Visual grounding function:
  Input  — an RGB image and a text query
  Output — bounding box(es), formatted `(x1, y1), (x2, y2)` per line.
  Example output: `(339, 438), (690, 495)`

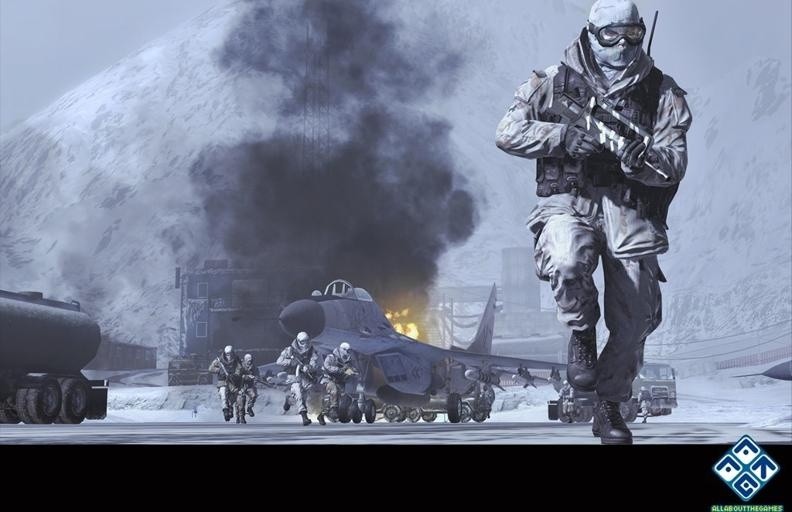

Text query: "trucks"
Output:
(552, 362), (677, 428)
(0, 288), (101, 425)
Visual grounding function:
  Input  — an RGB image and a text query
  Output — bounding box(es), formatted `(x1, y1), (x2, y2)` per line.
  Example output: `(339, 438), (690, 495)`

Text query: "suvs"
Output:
(168, 359), (212, 387)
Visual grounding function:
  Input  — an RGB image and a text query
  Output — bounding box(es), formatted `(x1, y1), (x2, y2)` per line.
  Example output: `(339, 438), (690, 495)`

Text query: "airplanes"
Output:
(254, 279), (571, 424)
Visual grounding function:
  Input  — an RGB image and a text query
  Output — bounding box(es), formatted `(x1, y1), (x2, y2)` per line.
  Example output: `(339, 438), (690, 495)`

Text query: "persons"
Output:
(318, 343), (360, 425)
(238, 354), (260, 423)
(558, 379), (574, 423)
(275, 332), (319, 425)
(492, 0), (692, 444)
(520, 366), (537, 388)
(210, 345), (244, 424)
(638, 386), (652, 424)
